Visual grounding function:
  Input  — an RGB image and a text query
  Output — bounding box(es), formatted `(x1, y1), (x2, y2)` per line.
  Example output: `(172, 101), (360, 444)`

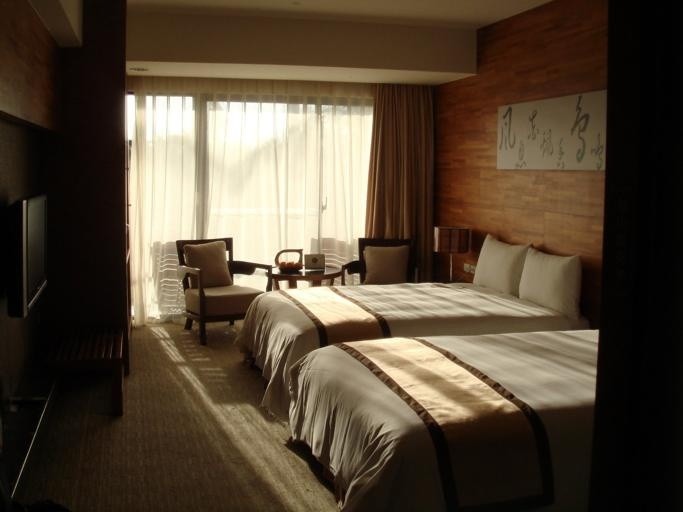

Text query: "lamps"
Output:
(433, 227), (470, 282)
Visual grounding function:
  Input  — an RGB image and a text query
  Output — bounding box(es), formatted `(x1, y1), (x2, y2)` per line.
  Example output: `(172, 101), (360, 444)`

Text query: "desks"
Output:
(24, 330), (124, 418)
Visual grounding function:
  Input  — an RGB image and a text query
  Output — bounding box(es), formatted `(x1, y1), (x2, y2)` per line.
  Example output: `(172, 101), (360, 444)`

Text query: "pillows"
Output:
(363, 245), (410, 284)
(182, 240), (233, 288)
(473, 234), (583, 323)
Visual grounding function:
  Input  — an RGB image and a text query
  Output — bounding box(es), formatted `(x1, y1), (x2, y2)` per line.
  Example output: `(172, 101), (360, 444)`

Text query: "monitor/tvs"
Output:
(4, 192), (47, 317)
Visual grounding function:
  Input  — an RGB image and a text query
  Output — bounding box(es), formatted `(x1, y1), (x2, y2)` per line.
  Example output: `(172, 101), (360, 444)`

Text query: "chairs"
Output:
(342, 238), (418, 286)
(176, 238), (272, 345)
